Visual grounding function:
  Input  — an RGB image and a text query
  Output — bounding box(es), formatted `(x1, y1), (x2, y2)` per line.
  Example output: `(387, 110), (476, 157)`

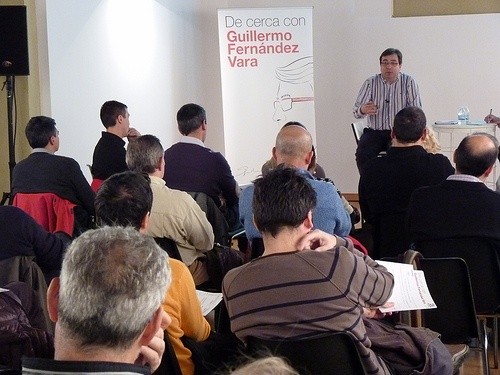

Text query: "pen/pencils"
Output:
(487, 108), (492, 123)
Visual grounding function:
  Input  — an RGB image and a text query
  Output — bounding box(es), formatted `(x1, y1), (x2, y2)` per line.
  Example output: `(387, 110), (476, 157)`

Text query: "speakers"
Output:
(0, 5), (29, 75)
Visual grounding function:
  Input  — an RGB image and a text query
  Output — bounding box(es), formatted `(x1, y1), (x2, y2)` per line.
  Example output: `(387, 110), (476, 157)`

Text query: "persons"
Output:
(227, 356), (300, 375)
(21, 226), (171, 374)
(407, 132), (500, 314)
(308, 146), (360, 225)
(94, 174), (212, 375)
(10, 116), (97, 237)
(221, 163), (469, 374)
(353, 48), (425, 174)
(0, 205), (74, 336)
(239, 125), (351, 259)
(127, 134), (248, 289)
(261, 121), (326, 179)
(485, 115), (500, 127)
(91, 100), (141, 183)
(358, 105), (456, 259)
(163, 104), (249, 253)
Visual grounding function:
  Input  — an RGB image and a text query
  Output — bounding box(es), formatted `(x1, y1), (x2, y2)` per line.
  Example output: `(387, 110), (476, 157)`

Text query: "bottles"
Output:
(458, 106), (466, 126)
(464, 106), (470, 125)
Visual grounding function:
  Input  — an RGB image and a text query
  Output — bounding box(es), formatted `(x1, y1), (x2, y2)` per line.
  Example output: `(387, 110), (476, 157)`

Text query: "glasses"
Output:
(380, 61), (399, 67)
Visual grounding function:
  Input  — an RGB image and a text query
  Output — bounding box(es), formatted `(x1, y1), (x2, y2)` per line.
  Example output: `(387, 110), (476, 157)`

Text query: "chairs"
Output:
(73, 183), (500, 375)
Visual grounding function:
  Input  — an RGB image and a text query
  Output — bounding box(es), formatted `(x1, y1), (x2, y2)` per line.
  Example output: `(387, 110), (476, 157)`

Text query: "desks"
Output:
(433, 121), (497, 191)
